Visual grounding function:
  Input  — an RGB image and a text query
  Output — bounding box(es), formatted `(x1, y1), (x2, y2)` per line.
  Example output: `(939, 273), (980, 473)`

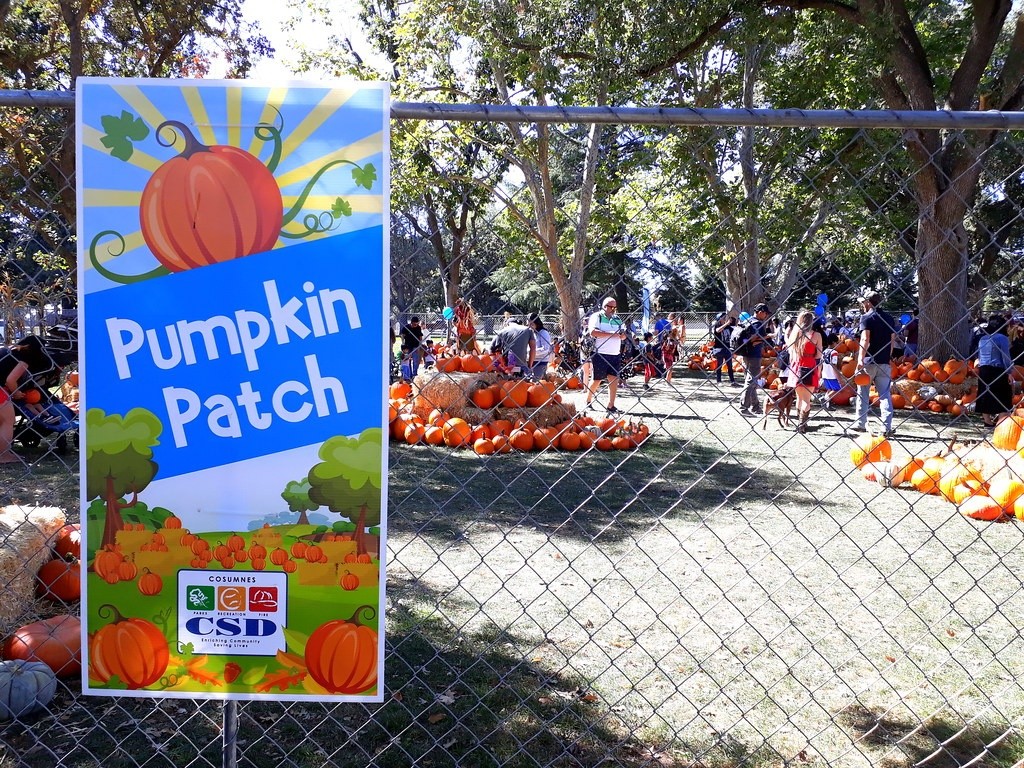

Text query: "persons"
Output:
(787, 312), (822, 433)
(849, 291), (896, 437)
(971, 315), (1024, 367)
(713, 311), (740, 386)
(820, 333), (841, 411)
(581, 297), (685, 414)
(978, 315), (1014, 428)
(525, 313), (551, 382)
(402, 317), (435, 376)
(893, 310), (918, 359)
(740, 303), (776, 416)
(491, 318), (536, 378)
(768, 317), (797, 345)
(0, 335), (61, 463)
(446, 310), (481, 358)
(812, 315), (854, 337)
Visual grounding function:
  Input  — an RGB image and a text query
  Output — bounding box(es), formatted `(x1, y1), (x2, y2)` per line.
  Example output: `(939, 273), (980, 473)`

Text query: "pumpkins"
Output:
(65, 369), (79, 387)
(23, 389), (41, 404)
(687, 340), (1024, 522)
(0, 523), (82, 722)
(389, 341), (649, 451)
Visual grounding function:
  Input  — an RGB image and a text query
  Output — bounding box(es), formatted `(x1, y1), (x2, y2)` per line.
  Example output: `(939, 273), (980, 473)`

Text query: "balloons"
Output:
(740, 312), (750, 321)
(443, 308), (452, 320)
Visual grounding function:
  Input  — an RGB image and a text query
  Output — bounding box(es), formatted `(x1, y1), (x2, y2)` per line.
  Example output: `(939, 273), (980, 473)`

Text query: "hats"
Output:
(754, 303), (772, 314)
(739, 312), (750, 322)
(716, 313), (726, 321)
(857, 291), (879, 303)
(653, 312), (660, 319)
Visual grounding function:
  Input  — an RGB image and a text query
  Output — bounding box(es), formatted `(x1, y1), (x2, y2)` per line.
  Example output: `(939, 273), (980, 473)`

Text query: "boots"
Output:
(796, 407), (811, 432)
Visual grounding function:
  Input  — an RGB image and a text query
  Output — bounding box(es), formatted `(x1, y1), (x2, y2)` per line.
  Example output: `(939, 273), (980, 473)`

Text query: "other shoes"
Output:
(642, 384), (653, 391)
(850, 426), (866, 432)
(882, 428), (895, 438)
(731, 382), (741, 388)
(587, 402), (593, 410)
(741, 409), (756, 417)
(607, 406), (624, 414)
(817, 392), (826, 407)
(39, 413), (60, 425)
(717, 381), (724, 386)
(0, 453), (24, 463)
(752, 407), (763, 414)
(984, 422), (995, 427)
(824, 406), (836, 411)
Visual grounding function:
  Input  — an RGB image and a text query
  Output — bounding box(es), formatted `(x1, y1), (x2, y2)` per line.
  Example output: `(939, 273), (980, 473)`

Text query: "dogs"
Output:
(763, 386), (796, 430)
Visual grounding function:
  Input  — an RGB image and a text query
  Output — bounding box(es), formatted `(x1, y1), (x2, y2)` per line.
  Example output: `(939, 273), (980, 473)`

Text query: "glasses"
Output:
(606, 305), (617, 310)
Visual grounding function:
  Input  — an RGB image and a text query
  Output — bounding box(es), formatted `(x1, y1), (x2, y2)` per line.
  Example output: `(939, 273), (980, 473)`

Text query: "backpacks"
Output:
(777, 350), (790, 371)
(729, 317), (761, 355)
